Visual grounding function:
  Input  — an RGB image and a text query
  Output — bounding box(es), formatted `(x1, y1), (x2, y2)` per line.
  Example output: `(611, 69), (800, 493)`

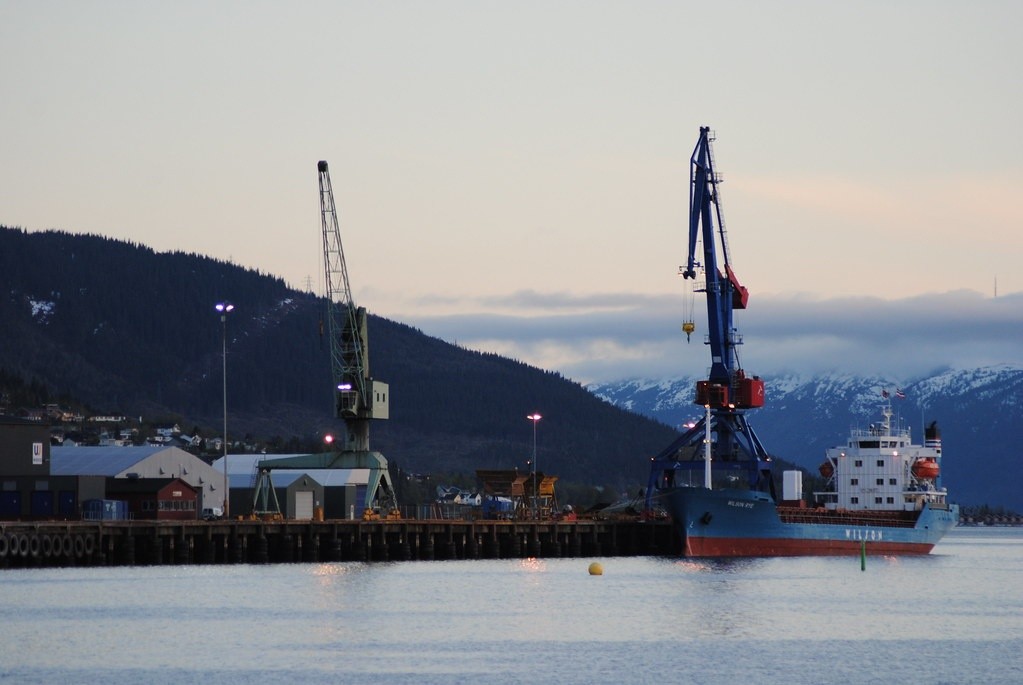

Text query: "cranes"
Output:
(245, 159), (403, 522)
(645, 124), (777, 518)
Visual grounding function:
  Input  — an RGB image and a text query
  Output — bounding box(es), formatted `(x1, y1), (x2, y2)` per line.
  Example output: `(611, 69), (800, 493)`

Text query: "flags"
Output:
(896, 388), (906, 400)
(881, 390), (890, 399)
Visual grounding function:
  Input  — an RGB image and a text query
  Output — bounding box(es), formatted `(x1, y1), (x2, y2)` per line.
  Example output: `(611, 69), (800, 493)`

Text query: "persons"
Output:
(562, 502), (574, 515)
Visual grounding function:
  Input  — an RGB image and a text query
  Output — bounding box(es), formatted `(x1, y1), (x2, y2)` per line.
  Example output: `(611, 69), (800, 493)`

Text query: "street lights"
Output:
(216, 303), (235, 521)
(526, 415), (541, 508)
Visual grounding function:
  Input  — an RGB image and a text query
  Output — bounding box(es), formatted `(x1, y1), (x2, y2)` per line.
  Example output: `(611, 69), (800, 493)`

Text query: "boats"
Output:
(913, 458), (939, 479)
(677, 386), (961, 560)
(819, 461), (838, 479)
